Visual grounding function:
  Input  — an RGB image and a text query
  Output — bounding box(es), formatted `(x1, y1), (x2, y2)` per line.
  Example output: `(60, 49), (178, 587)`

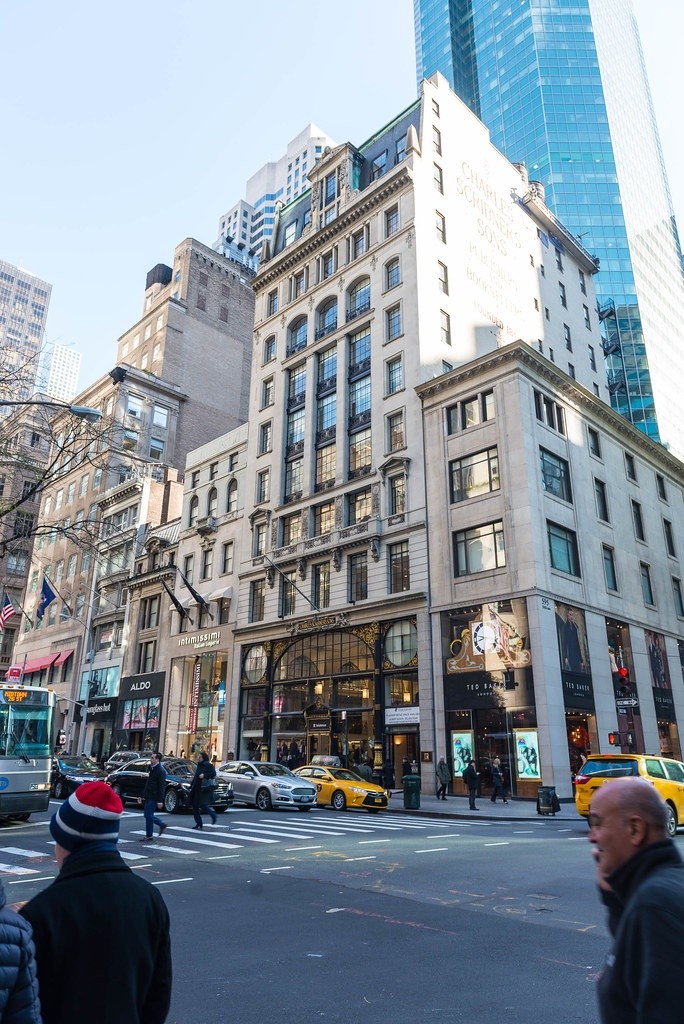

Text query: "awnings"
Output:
(169, 587), (233, 611)
(4, 649), (74, 677)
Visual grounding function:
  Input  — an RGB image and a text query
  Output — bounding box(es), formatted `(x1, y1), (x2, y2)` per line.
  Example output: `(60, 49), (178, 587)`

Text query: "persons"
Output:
(180, 745), (183, 757)
(132, 698), (160, 723)
(60, 748), (109, 769)
(16, 780), (172, 1024)
(137, 752), (168, 842)
(649, 631), (669, 689)
(0, 868), (42, 1024)
(246, 737), (258, 756)
(189, 751), (218, 829)
(276, 752), (294, 771)
(281, 737), (307, 759)
(588, 776), (684, 1024)
(522, 741), (538, 775)
(562, 607), (585, 673)
(382, 756), (509, 811)
(457, 743), (471, 772)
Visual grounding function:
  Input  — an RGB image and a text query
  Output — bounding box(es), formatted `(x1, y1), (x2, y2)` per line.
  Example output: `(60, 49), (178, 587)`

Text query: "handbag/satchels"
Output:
(502, 778), (510, 794)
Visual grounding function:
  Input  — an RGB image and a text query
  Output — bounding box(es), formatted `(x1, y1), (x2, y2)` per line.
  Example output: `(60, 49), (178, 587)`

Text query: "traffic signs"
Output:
(616, 698), (638, 716)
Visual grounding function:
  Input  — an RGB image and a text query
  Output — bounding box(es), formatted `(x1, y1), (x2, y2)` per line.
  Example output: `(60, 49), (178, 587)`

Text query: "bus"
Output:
(0, 682), (56, 823)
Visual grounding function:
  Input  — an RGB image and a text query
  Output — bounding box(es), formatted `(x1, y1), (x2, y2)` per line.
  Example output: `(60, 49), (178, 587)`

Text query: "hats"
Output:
(49, 783), (124, 852)
(440, 756), (445, 761)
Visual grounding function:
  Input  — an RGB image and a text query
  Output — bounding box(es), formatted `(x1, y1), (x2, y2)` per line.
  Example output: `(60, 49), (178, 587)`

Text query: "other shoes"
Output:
(442, 797), (448, 801)
(491, 798), (496, 803)
(139, 836), (153, 842)
(503, 799), (508, 804)
(470, 807), (480, 811)
(436, 793), (440, 800)
(158, 824), (168, 836)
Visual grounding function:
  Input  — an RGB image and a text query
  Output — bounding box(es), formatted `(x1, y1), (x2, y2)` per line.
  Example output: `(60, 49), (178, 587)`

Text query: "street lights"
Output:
(60, 614), (93, 756)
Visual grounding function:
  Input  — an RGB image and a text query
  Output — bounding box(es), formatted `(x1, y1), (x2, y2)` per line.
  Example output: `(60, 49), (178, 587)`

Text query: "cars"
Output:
(51, 757), (108, 795)
(214, 761), (318, 812)
(104, 755), (234, 814)
(289, 755), (389, 814)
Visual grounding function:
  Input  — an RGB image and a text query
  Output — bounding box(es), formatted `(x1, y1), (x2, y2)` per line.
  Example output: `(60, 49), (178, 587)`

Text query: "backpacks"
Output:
(463, 769), (470, 785)
(201, 766), (219, 791)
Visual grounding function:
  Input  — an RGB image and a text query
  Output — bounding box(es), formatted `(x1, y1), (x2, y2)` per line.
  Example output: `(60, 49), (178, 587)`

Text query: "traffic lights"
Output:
(609, 733), (620, 745)
(625, 734), (634, 744)
(619, 666), (631, 695)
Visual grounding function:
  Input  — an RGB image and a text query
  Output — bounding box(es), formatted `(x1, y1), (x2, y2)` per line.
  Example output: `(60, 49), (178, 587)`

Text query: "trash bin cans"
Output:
(401, 775), (421, 809)
(537, 786), (555, 816)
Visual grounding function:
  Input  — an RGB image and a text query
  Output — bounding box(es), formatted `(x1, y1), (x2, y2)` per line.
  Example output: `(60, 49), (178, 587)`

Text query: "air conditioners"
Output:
(195, 517), (215, 535)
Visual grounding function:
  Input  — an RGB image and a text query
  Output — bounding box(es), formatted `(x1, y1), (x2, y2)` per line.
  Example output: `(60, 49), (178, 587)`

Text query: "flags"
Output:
(0, 589), (17, 632)
(34, 575), (56, 631)
(158, 572), (205, 618)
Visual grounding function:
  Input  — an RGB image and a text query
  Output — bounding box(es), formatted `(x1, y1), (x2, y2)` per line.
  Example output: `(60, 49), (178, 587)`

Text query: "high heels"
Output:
(192, 823), (202, 829)
(211, 813), (218, 825)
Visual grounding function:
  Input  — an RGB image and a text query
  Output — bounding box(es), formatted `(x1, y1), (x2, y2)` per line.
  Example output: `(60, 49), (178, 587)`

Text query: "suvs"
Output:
(575, 753), (684, 836)
(104, 750), (154, 773)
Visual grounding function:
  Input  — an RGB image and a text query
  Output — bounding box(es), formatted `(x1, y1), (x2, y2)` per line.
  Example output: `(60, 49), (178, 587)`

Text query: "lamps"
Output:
(349, 600), (356, 606)
(278, 615), (284, 620)
